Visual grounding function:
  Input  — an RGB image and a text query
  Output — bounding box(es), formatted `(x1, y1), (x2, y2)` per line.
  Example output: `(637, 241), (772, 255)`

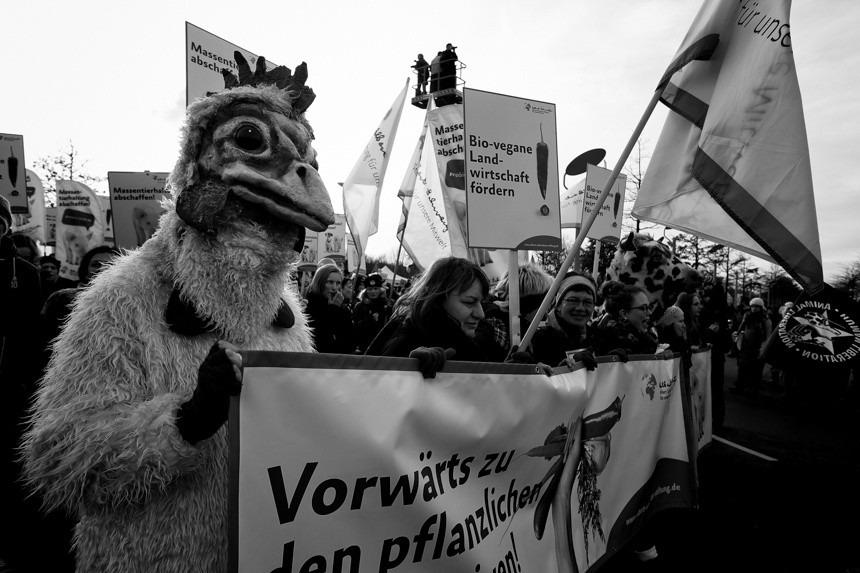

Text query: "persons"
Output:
(365, 256), (534, 379)
(18, 51), (336, 573)
(531, 271), (598, 371)
(0, 196), (124, 383)
(307, 257), (411, 356)
(483, 262), (554, 362)
(594, 273), (794, 428)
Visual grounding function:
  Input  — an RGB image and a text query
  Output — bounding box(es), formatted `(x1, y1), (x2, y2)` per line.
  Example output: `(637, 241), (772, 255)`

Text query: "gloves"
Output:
(609, 348), (631, 364)
(409, 346), (456, 377)
(574, 346), (598, 371)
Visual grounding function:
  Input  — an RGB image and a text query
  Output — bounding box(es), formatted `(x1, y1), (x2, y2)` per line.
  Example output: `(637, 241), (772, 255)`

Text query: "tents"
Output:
(369, 266), (408, 288)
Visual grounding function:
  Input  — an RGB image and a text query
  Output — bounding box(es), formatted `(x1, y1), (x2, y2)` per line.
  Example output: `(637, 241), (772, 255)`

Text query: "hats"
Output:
(0, 195), (12, 227)
(556, 275), (596, 307)
(750, 298), (764, 307)
(364, 274), (382, 288)
(653, 306), (684, 327)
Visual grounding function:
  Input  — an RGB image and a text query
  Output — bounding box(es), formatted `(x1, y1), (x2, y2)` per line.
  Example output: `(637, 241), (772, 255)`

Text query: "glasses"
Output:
(632, 304), (651, 315)
(562, 298), (593, 307)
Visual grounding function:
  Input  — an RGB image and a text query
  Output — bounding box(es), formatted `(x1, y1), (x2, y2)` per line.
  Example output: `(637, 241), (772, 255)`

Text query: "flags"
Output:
(630, 0), (825, 300)
(338, 77), (413, 267)
(397, 98), (494, 271)
